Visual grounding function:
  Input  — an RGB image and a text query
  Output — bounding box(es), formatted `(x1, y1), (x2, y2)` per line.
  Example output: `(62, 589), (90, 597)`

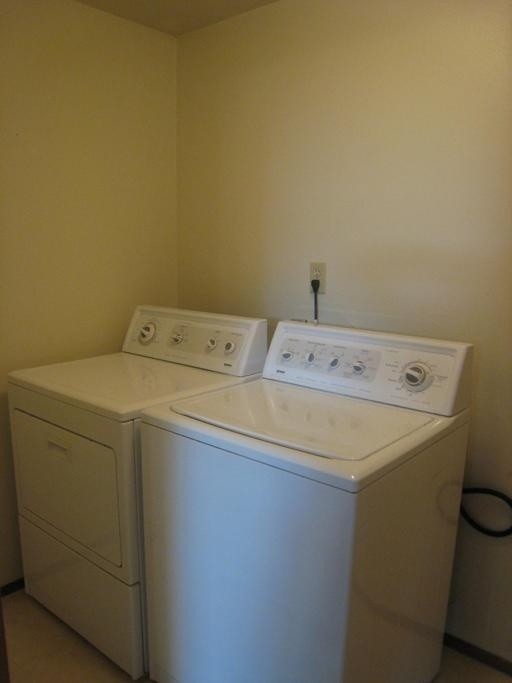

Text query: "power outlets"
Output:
(309, 263), (323, 290)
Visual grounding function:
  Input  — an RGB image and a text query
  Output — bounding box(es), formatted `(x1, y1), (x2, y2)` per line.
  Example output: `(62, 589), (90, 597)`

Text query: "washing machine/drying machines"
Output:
(140, 319), (476, 683)
(7, 306), (269, 683)
